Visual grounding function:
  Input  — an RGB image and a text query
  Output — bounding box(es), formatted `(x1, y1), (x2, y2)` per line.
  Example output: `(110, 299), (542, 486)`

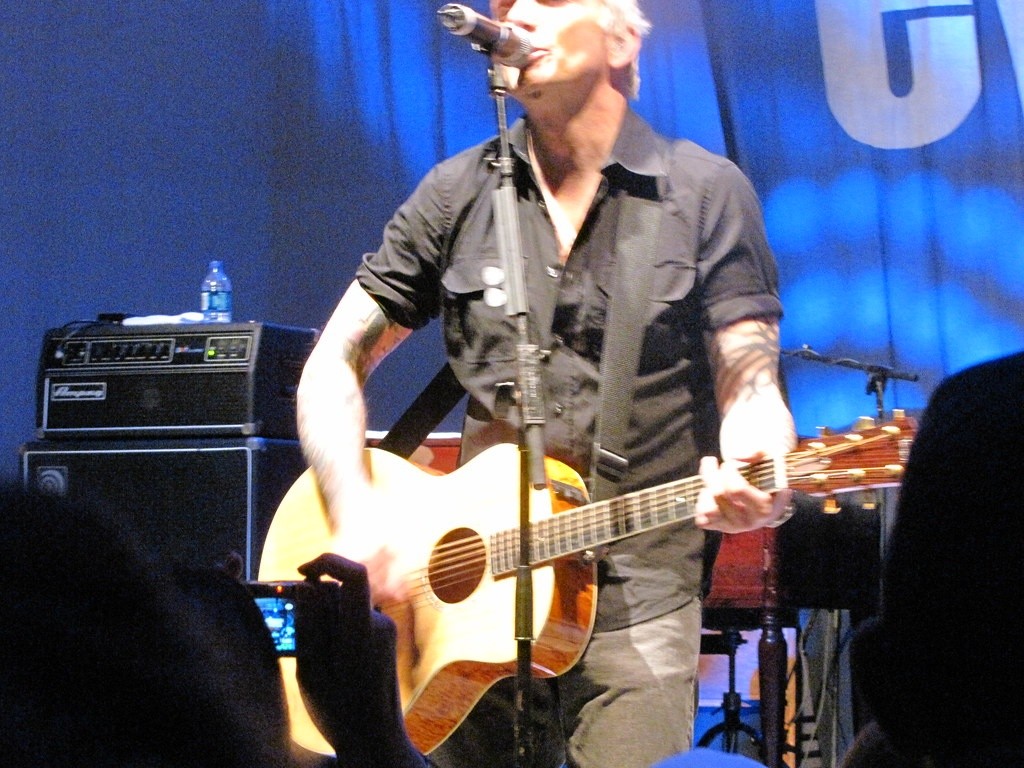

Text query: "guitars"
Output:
(251, 404), (916, 763)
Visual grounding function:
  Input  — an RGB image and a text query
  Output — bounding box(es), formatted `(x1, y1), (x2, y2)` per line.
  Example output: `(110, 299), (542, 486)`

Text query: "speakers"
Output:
(13, 437), (308, 768)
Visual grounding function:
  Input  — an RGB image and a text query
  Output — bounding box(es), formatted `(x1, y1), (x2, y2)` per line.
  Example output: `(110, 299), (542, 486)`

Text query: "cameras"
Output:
(231, 577), (341, 656)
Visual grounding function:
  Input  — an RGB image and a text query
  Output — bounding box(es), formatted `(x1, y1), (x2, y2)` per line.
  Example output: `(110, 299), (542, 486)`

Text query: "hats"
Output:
(0, 477), (289, 768)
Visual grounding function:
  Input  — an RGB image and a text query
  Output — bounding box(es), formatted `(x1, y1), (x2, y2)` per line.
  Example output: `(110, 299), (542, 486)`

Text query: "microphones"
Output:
(435, 3), (531, 68)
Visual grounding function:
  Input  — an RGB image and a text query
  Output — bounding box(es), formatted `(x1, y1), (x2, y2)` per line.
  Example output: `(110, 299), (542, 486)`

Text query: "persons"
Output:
(0, 476), (438, 768)
(294, 0), (800, 768)
(831, 350), (1024, 768)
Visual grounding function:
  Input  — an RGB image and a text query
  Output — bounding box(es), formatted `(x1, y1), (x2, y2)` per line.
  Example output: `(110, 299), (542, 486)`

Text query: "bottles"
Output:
(201, 261), (233, 323)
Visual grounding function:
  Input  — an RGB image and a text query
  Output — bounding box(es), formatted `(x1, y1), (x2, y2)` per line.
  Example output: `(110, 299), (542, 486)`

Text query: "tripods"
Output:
(696, 627), (768, 762)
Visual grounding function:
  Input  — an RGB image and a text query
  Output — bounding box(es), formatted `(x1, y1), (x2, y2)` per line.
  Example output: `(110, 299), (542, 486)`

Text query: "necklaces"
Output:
(526, 133), (572, 247)
